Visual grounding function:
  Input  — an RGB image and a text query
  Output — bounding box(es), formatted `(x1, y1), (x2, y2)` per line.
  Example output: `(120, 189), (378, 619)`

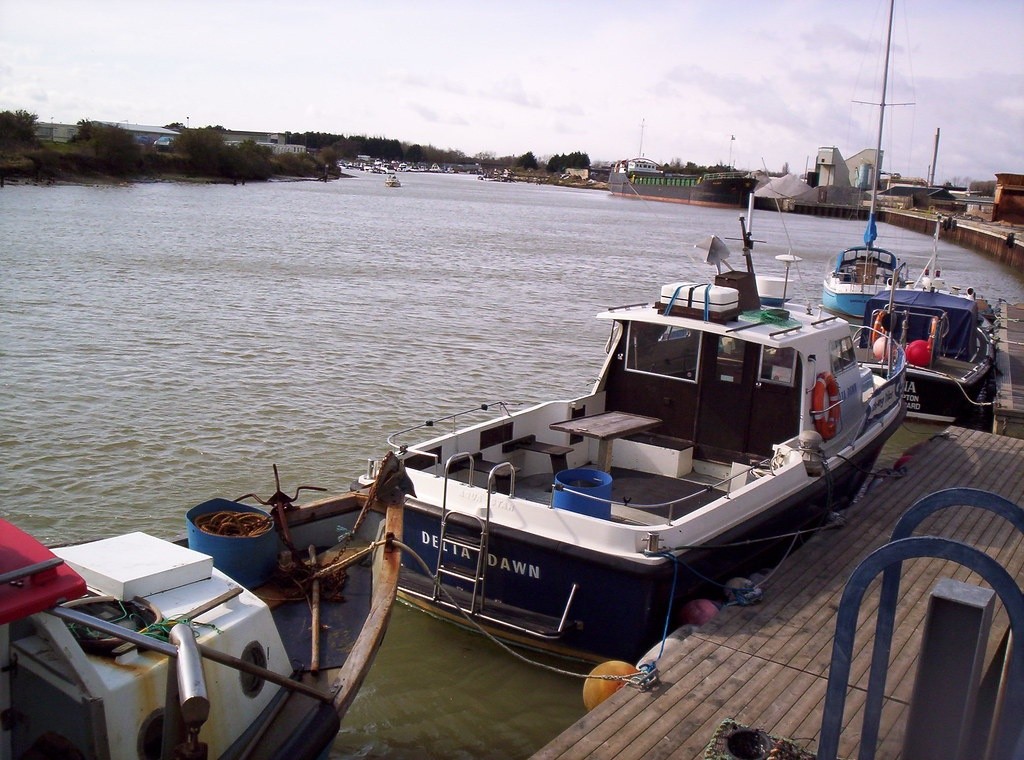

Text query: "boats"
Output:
(355, 190), (910, 668)
(0, 450), (417, 760)
(850, 213), (999, 429)
(385, 175), (402, 188)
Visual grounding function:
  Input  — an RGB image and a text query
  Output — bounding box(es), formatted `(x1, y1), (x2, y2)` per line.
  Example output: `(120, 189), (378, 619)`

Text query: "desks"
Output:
(549, 410), (662, 475)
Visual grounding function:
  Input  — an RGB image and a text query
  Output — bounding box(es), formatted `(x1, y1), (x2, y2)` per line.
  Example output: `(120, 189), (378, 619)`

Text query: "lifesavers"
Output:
(872, 311), (895, 345)
(929, 317), (938, 349)
(812, 372), (842, 440)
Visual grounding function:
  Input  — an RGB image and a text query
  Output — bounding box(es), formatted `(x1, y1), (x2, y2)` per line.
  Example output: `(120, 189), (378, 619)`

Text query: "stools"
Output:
(517, 440), (574, 480)
(463, 459), (521, 496)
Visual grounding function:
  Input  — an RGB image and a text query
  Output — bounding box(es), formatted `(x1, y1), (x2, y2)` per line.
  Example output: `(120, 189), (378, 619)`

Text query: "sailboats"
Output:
(821, 0), (913, 320)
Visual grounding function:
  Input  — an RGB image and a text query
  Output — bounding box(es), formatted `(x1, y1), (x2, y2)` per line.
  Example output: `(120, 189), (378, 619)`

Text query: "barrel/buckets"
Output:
(185, 498), (278, 591)
(552, 469), (613, 522)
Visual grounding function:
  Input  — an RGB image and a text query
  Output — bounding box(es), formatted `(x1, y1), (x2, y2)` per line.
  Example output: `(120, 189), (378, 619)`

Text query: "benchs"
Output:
(588, 432), (694, 479)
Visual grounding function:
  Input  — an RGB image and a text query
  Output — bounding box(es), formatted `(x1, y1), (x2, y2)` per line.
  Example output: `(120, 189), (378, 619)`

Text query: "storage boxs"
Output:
(661, 281), (740, 312)
